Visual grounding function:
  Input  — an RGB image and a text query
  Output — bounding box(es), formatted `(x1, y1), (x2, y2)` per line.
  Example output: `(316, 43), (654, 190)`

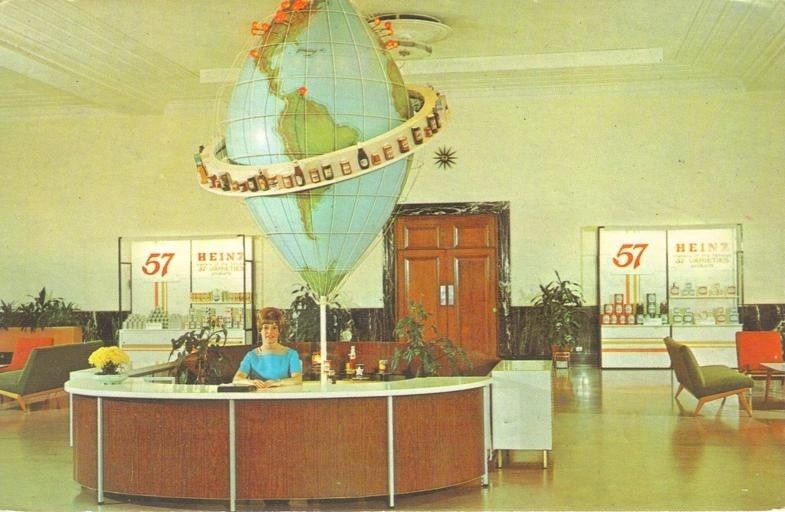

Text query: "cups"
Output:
(344, 362), (366, 379)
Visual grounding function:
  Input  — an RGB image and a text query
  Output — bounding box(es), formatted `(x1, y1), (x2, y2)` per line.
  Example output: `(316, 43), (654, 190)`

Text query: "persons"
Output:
(232, 306), (303, 388)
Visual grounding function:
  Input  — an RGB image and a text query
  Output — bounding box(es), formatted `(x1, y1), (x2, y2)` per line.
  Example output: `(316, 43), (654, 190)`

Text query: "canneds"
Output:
(207, 173), (294, 192)
(383, 145), (394, 160)
(397, 137), (410, 153)
(424, 114), (438, 137)
(309, 168), (320, 183)
(355, 363), (364, 379)
(601, 293), (667, 325)
(411, 126), (423, 144)
(339, 160), (352, 175)
(321, 164), (333, 180)
(378, 358), (389, 374)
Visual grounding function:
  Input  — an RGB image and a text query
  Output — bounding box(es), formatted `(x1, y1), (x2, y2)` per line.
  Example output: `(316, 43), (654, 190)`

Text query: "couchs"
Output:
(663, 336), (756, 417)
(0, 340), (104, 414)
(0, 337), (54, 372)
(735, 331), (785, 393)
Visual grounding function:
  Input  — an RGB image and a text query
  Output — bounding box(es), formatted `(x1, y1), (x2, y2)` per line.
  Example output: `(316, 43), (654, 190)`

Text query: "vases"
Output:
(94, 374), (128, 386)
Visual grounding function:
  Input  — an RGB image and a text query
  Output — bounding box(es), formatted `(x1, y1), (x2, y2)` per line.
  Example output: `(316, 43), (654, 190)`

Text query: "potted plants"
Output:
(530, 270), (587, 368)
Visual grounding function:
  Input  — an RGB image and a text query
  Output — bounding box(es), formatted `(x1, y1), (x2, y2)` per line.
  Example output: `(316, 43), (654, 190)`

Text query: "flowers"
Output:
(88, 346), (129, 373)
